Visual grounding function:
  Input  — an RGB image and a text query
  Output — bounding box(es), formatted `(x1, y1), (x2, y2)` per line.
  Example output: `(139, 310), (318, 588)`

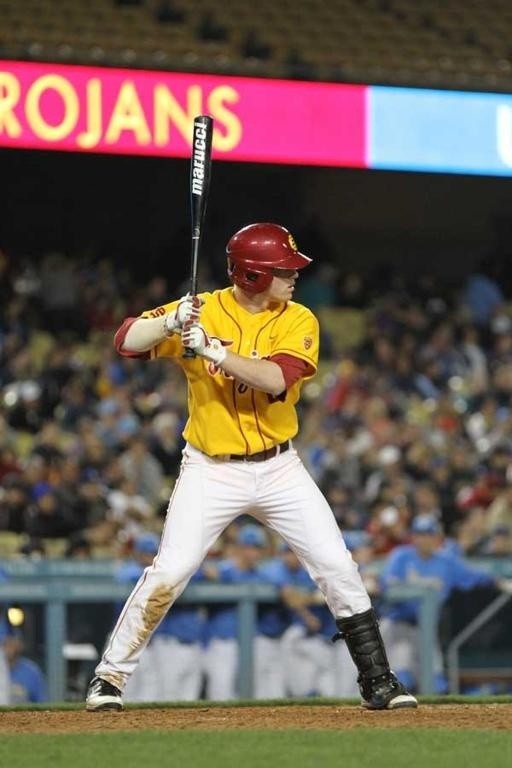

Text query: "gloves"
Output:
(182, 322), (227, 367)
(163, 294), (206, 339)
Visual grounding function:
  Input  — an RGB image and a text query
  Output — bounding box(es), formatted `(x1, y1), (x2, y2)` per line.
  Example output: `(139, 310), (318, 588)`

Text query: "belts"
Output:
(199, 438), (292, 463)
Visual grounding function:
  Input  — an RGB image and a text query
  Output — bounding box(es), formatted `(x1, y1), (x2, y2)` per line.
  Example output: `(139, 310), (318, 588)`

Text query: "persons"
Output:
(83, 223), (419, 711)
(3, 230), (509, 704)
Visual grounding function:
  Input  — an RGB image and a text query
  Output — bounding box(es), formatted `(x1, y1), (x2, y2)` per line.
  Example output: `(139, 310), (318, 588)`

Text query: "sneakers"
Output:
(361, 670), (420, 713)
(84, 676), (127, 711)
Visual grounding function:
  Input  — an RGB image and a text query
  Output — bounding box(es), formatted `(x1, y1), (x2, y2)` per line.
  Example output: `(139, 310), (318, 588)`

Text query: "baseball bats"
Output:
(183, 116), (213, 358)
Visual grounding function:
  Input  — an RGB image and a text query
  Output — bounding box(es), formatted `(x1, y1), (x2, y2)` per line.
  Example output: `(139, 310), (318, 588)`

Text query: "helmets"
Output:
(226, 222), (314, 294)
